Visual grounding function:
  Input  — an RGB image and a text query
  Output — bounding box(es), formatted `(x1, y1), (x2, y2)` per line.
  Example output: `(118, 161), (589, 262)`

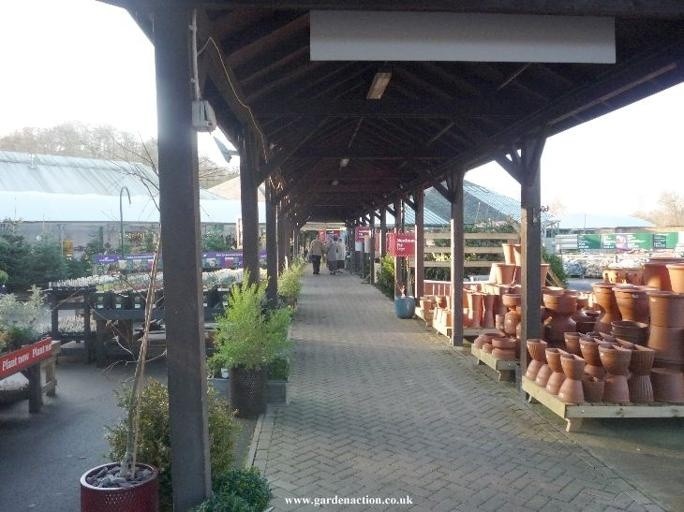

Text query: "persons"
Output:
(220, 234), (236, 250)
(289, 234), (349, 276)
(257, 231), (266, 249)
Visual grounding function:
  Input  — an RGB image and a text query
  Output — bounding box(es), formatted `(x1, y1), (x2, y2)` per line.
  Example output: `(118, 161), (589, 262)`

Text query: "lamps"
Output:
(366, 69), (391, 99)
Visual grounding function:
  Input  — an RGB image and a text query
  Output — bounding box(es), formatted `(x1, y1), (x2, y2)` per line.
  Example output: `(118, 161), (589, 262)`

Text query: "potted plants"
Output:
(277, 266), (302, 309)
(207, 265), (297, 418)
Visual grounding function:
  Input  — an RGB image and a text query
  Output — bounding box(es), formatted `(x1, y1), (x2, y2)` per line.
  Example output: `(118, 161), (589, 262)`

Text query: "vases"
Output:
(394, 297), (416, 318)
(81, 462), (160, 512)
